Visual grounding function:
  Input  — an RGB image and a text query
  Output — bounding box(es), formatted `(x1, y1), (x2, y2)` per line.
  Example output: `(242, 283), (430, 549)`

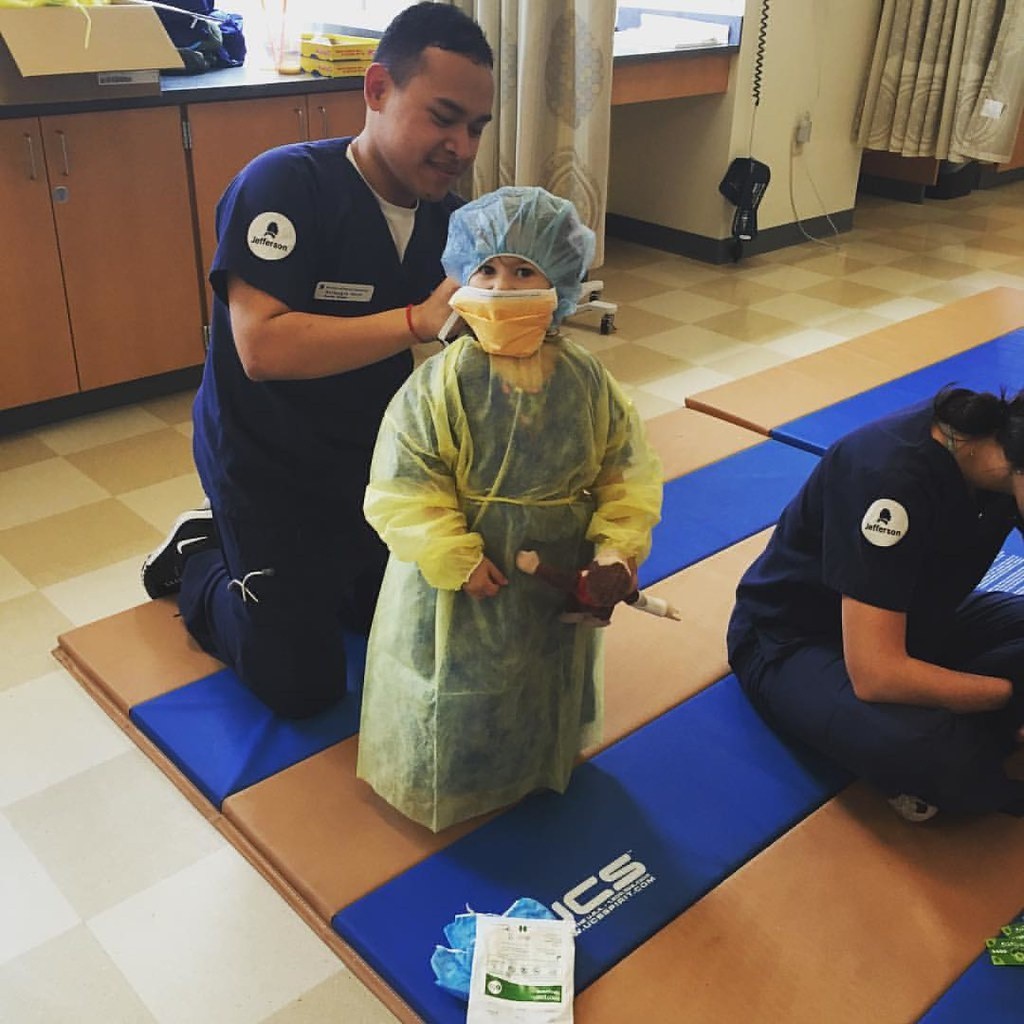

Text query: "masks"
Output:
(446, 284), (557, 358)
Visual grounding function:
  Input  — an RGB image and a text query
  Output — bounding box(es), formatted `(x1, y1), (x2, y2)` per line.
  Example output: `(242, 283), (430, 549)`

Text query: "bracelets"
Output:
(406, 303), (427, 345)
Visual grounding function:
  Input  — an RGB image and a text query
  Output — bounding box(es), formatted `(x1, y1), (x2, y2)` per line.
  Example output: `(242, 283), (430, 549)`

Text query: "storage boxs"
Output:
(0, 0), (185, 106)
(300, 33), (381, 78)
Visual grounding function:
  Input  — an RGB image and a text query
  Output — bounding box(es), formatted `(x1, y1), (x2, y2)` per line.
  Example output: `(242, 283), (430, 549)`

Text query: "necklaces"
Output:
(945, 425), (957, 451)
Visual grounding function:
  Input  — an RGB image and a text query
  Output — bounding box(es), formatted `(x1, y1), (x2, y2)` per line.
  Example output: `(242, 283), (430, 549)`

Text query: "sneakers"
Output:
(141, 508), (219, 600)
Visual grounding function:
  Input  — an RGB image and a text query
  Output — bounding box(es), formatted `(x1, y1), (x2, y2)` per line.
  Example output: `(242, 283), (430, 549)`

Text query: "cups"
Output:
(278, 37), (302, 76)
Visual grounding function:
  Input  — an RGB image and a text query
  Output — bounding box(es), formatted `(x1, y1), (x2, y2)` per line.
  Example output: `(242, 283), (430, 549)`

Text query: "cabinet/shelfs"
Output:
(0, 106), (207, 436)
(184, 89), (367, 339)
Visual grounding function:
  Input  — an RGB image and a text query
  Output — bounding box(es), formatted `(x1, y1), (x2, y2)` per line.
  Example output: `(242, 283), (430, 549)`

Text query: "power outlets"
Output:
(791, 124), (804, 156)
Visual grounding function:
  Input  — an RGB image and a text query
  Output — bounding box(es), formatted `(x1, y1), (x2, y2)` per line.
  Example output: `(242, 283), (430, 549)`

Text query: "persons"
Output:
(727, 382), (1024, 822)
(143, 1), (493, 714)
(362, 184), (663, 828)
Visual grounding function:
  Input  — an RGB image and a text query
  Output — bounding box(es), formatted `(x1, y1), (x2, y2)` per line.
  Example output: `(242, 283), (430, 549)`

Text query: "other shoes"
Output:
(888, 792), (938, 824)
